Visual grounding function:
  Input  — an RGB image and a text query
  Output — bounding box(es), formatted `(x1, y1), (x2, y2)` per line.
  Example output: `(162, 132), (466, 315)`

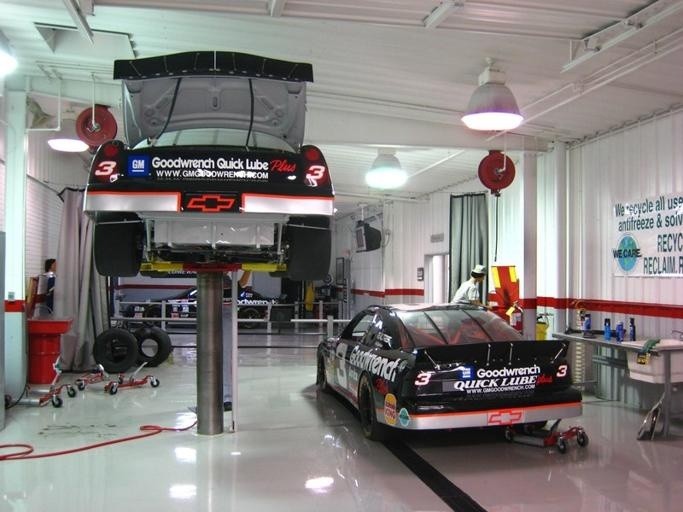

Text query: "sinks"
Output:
(622, 339), (683, 384)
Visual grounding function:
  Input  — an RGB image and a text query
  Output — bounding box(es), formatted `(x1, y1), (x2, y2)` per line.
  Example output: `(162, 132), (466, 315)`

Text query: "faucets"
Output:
(670, 329), (683, 341)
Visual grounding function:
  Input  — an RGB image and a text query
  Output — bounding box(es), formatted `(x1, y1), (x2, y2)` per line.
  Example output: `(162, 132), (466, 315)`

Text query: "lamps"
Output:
(461, 57), (524, 132)
(48, 109), (89, 152)
(366, 149), (407, 190)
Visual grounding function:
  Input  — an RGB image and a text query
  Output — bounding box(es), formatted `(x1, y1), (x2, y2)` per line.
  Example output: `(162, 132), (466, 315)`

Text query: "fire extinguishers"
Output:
(505, 301), (523, 335)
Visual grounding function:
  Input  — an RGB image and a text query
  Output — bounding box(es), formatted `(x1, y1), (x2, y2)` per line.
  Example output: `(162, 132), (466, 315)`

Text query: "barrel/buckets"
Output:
(28, 334), (60, 383)
(536, 315), (548, 340)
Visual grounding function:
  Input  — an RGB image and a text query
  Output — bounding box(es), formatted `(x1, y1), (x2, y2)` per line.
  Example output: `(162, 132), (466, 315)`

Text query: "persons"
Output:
(451, 264), (491, 310)
(44, 259), (56, 277)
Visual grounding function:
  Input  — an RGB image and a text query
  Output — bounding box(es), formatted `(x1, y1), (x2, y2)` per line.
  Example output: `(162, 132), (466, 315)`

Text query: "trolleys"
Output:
(0, 354), (76, 408)
(502, 415), (589, 455)
(74, 360), (160, 395)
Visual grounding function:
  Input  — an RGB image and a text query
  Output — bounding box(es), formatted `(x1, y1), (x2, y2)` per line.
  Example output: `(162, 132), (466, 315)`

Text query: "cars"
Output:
(315, 300), (581, 441)
(82, 49), (336, 282)
(123, 283), (277, 332)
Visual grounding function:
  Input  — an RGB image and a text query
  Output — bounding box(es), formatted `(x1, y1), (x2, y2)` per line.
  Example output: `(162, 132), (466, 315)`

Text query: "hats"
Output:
(472, 265), (488, 275)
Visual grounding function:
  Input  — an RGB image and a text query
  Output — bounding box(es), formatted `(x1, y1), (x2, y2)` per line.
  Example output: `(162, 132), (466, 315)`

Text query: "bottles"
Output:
(583, 313), (591, 333)
(629, 318), (636, 341)
(615, 321), (625, 343)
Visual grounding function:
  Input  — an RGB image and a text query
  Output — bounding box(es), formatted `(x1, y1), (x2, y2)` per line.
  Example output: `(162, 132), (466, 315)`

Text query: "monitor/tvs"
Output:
(355, 224), (380, 253)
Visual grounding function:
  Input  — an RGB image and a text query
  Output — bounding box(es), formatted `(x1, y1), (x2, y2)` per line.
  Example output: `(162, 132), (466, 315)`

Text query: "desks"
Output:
(551, 333), (683, 440)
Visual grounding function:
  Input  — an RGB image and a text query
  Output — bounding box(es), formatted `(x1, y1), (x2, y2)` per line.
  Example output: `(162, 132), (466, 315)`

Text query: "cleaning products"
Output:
(629, 318), (635, 341)
(616, 321), (623, 344)
